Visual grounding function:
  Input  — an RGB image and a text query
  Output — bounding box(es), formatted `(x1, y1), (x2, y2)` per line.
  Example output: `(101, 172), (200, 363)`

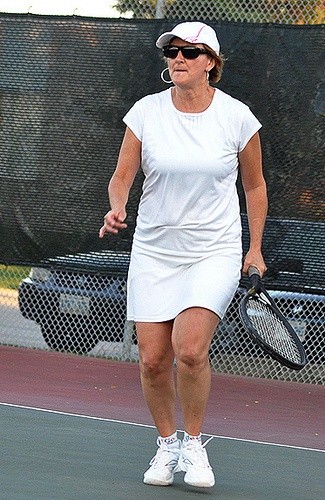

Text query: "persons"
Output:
(100, 20), (268, 488)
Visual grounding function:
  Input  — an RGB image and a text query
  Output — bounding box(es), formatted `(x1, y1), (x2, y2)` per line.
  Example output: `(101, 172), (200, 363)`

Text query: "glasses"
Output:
(161, 44), (213, 59)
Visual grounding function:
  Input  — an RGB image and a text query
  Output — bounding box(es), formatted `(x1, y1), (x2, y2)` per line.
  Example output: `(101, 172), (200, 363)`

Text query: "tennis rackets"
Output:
(239, 266), (307, 371)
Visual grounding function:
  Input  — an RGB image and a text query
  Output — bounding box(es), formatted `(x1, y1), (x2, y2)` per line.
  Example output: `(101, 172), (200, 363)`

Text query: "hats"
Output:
(156, 22), (220, 56)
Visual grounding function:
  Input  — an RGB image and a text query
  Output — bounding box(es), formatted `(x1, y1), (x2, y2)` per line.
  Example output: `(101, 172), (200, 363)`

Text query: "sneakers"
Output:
(179, 440), (215, 487)
(143, 440), (179, 486)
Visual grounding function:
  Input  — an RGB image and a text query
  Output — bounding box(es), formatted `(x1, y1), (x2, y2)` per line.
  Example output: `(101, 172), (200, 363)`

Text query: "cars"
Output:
(18, 214), (325, 364)
